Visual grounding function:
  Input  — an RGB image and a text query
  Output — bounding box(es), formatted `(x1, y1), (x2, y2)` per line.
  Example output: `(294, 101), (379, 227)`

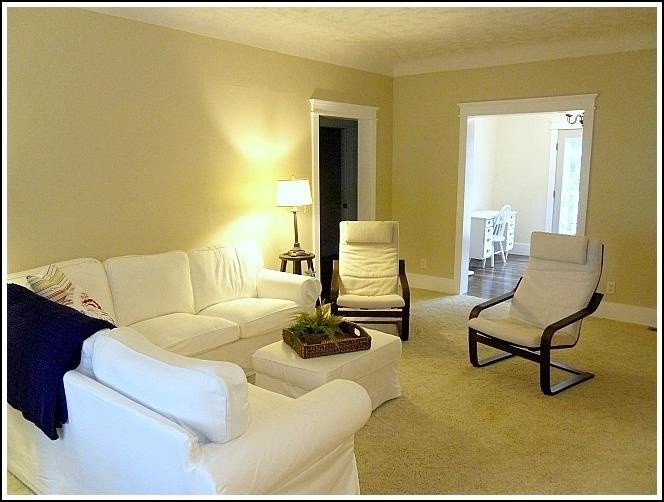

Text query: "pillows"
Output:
(26, 264), (116, 326)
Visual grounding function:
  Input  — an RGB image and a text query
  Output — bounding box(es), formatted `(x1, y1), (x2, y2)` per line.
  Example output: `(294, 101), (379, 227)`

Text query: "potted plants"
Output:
(283, 300), (346, 354)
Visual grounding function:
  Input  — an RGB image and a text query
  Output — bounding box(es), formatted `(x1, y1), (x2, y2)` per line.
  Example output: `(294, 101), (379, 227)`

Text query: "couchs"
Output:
(251, 326), (403, 414)
(7, 242), (322, 378)
(7, 283), (372, 493)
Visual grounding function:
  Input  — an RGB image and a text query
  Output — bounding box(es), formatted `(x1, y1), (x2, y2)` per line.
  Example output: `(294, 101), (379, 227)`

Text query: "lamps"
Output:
(274, 175), (314, 257)
(565, 111), (585, 127)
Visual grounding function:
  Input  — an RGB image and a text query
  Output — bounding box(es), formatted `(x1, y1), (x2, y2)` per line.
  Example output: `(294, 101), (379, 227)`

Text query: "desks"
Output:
(470, 210), (517, 268)
(279, 252), (321, 308)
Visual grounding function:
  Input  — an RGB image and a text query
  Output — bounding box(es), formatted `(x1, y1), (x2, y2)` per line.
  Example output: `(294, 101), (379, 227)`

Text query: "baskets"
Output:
(282, 321), (372, 358)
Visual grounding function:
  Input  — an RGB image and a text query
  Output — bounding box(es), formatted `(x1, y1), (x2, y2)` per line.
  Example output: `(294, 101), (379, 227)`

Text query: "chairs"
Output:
(466, 231), (605, 395)
(330, 220), (409, 340)
(491, 204), (511, 265)
